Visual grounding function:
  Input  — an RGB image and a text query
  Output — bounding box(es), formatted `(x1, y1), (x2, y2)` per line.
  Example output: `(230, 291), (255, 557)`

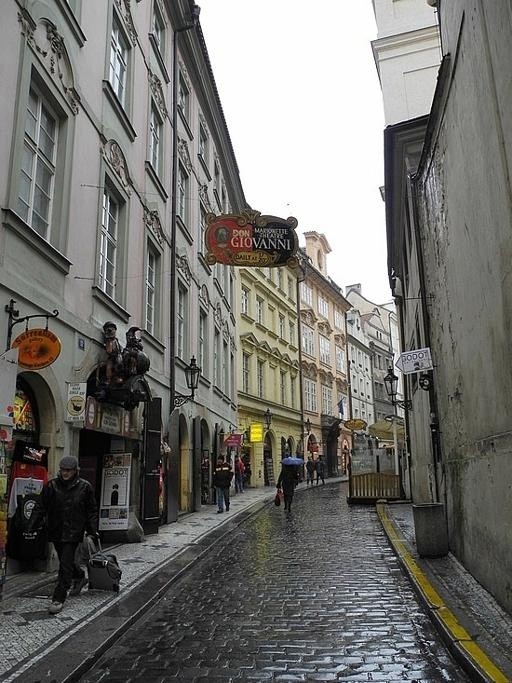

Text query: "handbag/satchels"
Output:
(274, 487), (282, 508)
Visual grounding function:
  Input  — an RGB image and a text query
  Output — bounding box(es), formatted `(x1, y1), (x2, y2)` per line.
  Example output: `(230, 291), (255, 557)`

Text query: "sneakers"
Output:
(48, 598), (64, 615)
(70, 577), (89, 597)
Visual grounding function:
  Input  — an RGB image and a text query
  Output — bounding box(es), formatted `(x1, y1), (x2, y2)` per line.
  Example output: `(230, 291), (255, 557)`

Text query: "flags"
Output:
(337, 400), (344, 416)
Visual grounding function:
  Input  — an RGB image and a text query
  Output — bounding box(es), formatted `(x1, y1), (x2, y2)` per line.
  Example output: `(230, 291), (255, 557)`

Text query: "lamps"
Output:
(383, 365), (413, 413)
(263, 407), (273, 430)
(303, 417), (313, 436)
(170, 355), (202, 407)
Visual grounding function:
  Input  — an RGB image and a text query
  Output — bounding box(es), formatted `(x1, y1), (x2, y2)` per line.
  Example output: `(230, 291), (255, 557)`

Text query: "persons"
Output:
(111, 484), (118, 506)
(28, 456), (99, 614)
(213, 455), (234, 513)
(235, 455), (245, 496)
(314, 457), (325, 485)
(276, 463), (298, 513)
(306, 456), (315, 484)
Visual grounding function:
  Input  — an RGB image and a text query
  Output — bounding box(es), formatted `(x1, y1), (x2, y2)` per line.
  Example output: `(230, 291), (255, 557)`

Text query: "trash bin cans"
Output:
(413, 503), (448, 559)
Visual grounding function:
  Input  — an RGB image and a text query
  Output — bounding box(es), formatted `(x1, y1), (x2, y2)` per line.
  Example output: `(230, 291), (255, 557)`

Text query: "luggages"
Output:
(85, 532), (121, 594)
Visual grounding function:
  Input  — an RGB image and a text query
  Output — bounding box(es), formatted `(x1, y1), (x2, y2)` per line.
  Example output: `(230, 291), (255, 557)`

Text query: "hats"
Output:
(59, 457), (78, 471)
